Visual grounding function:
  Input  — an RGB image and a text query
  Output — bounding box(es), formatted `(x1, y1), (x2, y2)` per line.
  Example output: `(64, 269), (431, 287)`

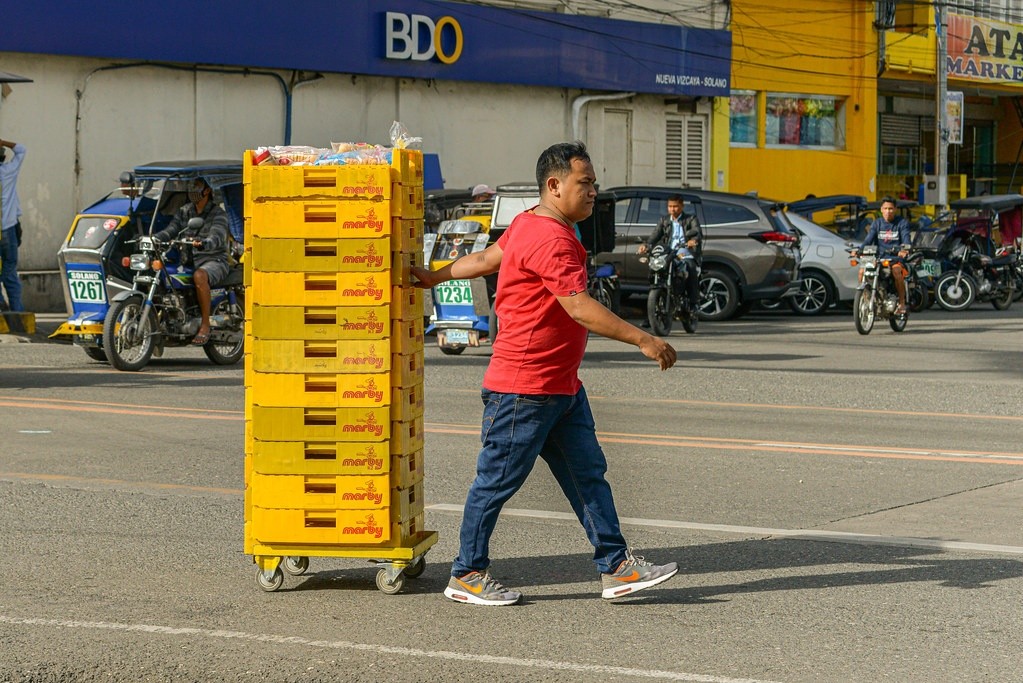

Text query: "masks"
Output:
(188, 190), (206, 205)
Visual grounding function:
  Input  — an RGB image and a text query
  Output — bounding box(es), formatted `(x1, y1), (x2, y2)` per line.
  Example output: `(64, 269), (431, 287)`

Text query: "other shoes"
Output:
(640, 319), (651, 329)
(690, 303), (700, 315)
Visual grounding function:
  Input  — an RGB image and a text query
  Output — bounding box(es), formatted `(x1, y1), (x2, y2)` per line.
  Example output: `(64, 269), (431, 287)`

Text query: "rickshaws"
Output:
(424, 182), (619, 355)
(48, 158), (244, 372)
(787, 193), (931, 253)
(908, 193), (1023, 312)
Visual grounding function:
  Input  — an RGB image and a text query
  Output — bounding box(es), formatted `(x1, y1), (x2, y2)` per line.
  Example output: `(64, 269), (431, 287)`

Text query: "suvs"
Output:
(596, 186), (802, 322)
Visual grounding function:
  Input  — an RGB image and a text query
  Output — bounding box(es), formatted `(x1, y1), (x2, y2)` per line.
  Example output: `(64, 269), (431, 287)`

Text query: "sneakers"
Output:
(601, 547), (680, 600)
(444, 564), (523, 606)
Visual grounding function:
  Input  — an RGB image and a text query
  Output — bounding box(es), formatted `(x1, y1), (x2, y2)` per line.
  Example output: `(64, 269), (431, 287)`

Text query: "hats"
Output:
(472, 184), (496, 196)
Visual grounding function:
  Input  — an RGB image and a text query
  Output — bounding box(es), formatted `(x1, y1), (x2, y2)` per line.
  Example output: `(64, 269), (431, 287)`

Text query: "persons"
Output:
(850, 198), (912, 314)
(464, 184), (497, 216)
(152, 177), (230, 345)
(0, 139), (26, 312)
(800, 193), (817, 221)
(573, 222), (581, 241)
(638, 194), (704, 327)
(410, 143), (679, 607)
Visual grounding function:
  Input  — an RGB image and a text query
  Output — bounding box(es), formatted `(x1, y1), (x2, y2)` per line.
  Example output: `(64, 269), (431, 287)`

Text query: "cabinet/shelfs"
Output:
(243, 149), (423, 547)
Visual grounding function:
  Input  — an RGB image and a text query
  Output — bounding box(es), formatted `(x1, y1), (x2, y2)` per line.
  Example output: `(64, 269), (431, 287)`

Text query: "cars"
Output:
(783, 211), (866, 316)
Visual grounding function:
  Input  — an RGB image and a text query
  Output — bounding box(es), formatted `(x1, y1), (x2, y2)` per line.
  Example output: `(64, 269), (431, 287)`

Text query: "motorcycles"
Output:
(634, 230), (698, 336)
(844, 237), (922, 334)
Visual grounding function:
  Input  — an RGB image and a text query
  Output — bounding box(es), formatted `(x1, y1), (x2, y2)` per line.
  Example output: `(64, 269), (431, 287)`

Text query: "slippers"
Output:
(894, 304), (911, 315)
(191, 327), (214, 346)
